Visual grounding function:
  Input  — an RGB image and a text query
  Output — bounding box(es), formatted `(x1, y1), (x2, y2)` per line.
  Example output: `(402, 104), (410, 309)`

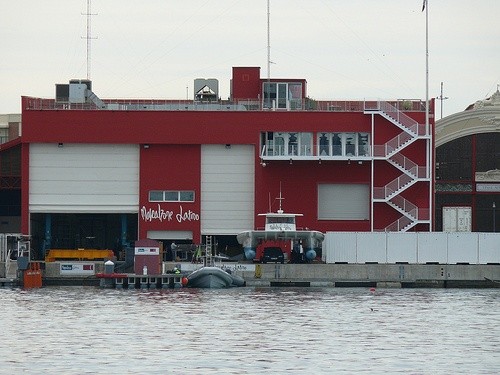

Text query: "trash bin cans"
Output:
(23, 261), (46, 290)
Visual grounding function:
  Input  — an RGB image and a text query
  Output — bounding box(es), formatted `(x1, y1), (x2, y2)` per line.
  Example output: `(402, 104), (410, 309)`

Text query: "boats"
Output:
(182, 234), (245, 290)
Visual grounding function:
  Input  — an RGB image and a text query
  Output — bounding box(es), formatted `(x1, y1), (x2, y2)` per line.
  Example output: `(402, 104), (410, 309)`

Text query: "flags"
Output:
(422, 0), (426, 12)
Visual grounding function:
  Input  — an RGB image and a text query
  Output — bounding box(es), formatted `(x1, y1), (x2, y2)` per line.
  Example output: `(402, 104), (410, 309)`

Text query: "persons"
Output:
(170, 240), (179, 263)
(190, 245), (202, 264)
